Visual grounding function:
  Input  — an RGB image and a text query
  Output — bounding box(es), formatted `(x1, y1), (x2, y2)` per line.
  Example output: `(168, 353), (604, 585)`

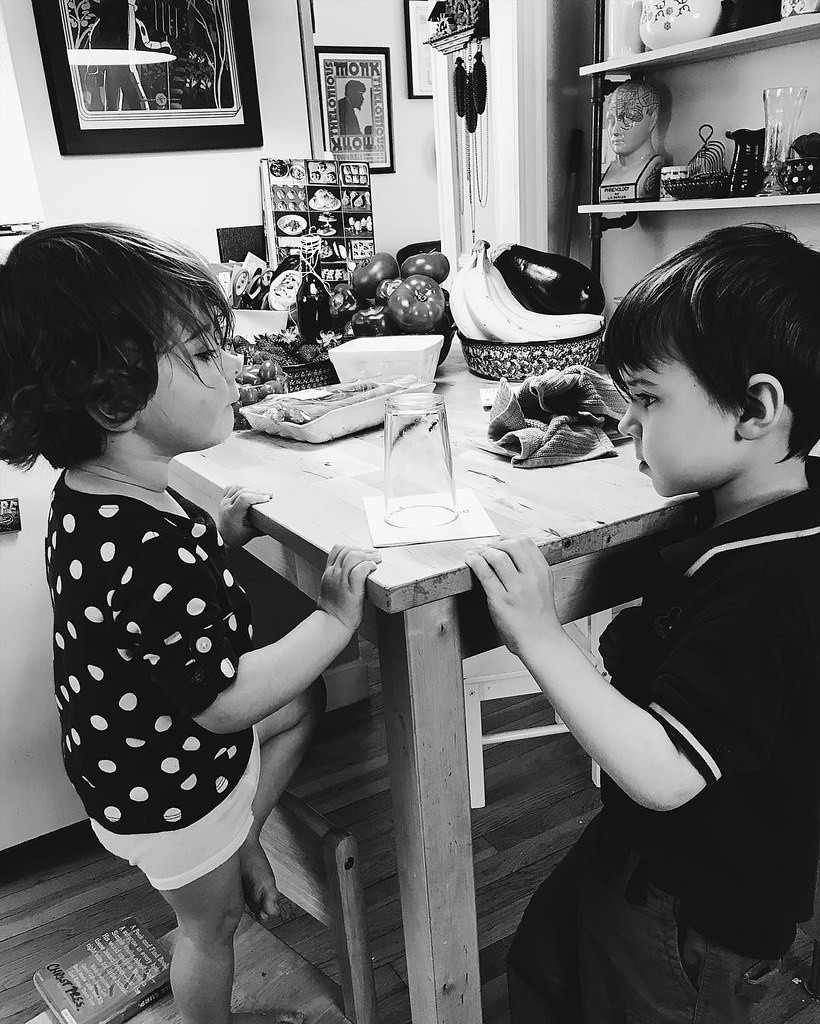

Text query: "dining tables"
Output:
(166, 336), (702, 1024)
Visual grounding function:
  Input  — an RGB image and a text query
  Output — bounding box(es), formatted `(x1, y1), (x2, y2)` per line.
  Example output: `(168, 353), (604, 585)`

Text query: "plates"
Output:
(309, 197), (342, 210)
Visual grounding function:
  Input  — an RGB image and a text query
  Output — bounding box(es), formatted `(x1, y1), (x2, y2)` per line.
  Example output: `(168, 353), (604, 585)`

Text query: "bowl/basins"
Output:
(328, 335), (444, 383)
(282, 359), (341, 393)
(277, 214), (307, 235)
(457, 321), (608, 383)
(421, 326), (456, 367)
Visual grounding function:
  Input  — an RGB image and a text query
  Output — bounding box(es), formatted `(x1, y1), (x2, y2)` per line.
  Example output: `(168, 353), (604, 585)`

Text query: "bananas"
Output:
(450, 240), (606, 339)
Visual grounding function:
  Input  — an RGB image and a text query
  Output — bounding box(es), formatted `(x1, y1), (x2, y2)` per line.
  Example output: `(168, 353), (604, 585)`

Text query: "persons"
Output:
(465, 222), (820, 1024)
(0, 223), (381, 1024)
(599, 81), (663, 202)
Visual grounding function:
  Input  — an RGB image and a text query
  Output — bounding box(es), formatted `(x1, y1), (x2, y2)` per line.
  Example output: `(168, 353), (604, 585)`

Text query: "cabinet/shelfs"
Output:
(578, 13), (820, 213)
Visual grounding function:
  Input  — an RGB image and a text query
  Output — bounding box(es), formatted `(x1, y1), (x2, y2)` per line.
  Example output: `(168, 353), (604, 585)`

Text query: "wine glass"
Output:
(755, 86), (808, 197)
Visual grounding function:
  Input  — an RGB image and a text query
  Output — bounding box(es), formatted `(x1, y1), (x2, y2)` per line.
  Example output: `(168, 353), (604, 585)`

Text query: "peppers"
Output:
(330, 278), (405, 335)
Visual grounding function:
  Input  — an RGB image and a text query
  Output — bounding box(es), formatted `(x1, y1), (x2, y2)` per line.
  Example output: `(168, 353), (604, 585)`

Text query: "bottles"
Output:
(296, 234), (331, 345)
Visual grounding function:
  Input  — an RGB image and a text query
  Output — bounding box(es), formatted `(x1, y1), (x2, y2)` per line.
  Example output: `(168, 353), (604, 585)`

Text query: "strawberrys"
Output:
(220, 330), (341, 371)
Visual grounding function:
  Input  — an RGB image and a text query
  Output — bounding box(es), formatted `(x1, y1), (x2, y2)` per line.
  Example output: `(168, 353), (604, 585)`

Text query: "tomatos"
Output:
(227, 361), (287, 402)
(388, 275), (445, 333)
(352, 252), (399, 299)
(401, 251), (449, 285)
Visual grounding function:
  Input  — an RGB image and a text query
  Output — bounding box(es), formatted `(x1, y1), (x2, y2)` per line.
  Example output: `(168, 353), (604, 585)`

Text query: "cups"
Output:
(776, 157), (820, 194)
(384, 393), (459, 529)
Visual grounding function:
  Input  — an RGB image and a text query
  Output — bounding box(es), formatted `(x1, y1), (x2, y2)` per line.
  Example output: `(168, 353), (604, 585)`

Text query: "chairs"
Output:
(24, 790), (380, 1024)
(462, 608), (612, 810)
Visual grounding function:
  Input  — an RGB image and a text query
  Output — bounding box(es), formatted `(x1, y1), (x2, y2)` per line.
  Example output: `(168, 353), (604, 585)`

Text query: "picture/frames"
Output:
(404, 0), (436, 99)
(31, 0), (264, 156)
(314, 46), (396, 174)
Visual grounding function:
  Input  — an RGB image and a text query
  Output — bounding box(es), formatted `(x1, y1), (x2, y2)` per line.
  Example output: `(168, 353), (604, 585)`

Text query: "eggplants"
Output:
(485, 238), (606, 319)
